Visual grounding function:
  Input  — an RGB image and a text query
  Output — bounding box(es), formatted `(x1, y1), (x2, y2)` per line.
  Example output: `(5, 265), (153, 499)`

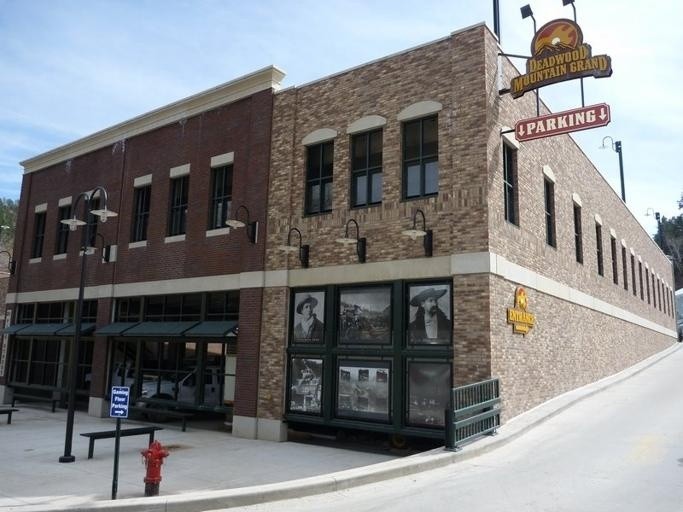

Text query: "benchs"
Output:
(80, 426), (163, 458)
(10, 392), (61, 412)
(128, 406), (194, 431)
(0, 408), (12, 424)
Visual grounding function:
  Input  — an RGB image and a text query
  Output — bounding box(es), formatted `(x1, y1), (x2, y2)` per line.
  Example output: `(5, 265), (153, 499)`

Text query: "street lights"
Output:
(58, 186), (118, 467)
(601, 134), (626, 205)
(646, 207), (663, 250)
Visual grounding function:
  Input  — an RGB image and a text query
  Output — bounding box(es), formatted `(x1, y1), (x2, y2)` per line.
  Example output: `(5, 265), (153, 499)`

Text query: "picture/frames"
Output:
(405, 360), (451, 430)
(339, 284), (392, 346)
(288, 352), (324, 417)
(336, 358), (392, 421)
(405, 281), (452, 346)
(290, 289), (326, 345)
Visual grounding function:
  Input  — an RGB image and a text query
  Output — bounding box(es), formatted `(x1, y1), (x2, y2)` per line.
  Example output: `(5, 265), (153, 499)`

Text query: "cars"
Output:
(87, 359), (223, 422)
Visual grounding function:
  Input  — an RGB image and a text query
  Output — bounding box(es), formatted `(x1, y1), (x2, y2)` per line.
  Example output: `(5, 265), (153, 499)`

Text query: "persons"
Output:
(409, 288), (450, 339)
(294, 293), (323, 342)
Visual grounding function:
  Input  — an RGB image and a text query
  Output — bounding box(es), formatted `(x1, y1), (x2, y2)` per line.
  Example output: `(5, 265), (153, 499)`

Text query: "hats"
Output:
(296, 293), (318, 314)
(410, 288), (448, 307)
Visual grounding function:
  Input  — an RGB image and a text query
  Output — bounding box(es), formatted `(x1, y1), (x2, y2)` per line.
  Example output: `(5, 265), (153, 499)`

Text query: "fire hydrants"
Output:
(142, 440), (170, 497)
